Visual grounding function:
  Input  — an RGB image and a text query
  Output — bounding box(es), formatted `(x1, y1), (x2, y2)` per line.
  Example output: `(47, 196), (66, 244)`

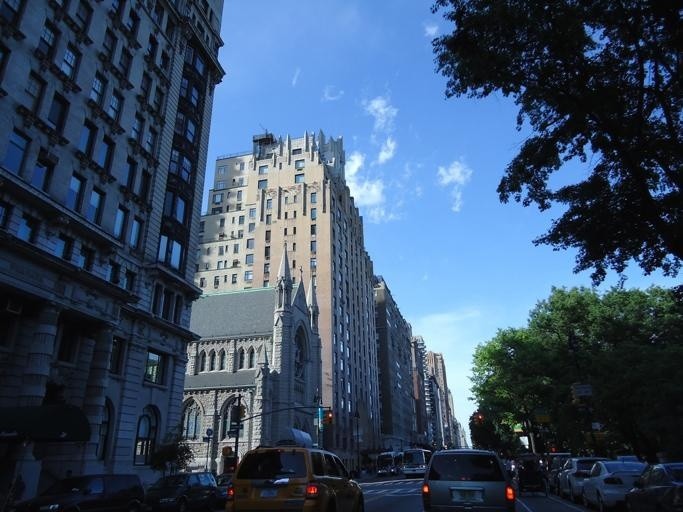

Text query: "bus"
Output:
(376, 452), (404, 478)
(401, 449), (432, 477)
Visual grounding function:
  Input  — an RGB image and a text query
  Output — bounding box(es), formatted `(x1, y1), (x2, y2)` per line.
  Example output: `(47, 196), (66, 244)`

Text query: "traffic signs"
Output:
(574, 383), (592, 397)
(230, 425), (242, 429)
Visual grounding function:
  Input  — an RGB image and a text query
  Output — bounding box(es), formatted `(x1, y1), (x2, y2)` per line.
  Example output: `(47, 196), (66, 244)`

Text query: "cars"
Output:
(422, 449), (515, 512)
(214, 473), (235, 500)
(145, 472), (218, 512)
(10, 474), (145, 512)
(225, 446), (364, 511)
(546, 453), (682, 512)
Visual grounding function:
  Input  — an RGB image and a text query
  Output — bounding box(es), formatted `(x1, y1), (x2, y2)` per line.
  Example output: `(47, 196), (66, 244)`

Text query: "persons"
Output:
(15, 473), (26, 502)
(66, 470), (73, 478)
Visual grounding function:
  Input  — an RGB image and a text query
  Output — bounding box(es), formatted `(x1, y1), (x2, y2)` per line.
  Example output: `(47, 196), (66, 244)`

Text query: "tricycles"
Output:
(516, 453), (548, 497)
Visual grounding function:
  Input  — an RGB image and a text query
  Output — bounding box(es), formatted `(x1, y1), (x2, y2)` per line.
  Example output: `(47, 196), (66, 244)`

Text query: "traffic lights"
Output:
(239, 406), (245, 418)
(328, 410), (332, 423)
(478, 415), (481, 420)
